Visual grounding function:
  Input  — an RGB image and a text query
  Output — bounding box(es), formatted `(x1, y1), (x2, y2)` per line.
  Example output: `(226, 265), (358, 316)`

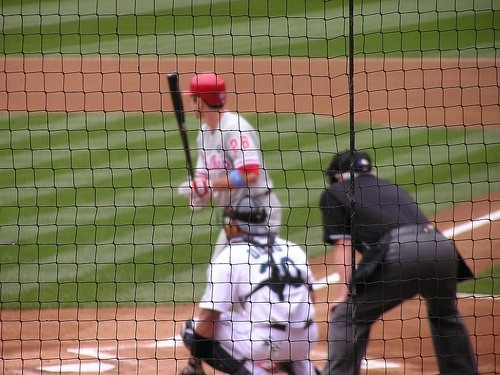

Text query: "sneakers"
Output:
(178, 364), (206, 375)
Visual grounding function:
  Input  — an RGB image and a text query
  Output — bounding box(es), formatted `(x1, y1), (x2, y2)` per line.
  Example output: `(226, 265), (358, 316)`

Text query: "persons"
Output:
(320, 150), (479, 375)
(183, 196), (322, 375)
(177, 71), (282, 374)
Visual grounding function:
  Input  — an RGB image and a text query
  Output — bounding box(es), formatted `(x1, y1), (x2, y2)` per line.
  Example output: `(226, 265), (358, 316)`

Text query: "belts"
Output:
(379, 223), (434, 245)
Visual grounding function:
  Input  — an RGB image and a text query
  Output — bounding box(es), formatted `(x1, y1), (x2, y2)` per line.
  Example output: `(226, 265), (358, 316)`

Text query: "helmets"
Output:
(179, 71), (226, 106)
(223, 196), (268, 234)
(327, 149), (372, 184)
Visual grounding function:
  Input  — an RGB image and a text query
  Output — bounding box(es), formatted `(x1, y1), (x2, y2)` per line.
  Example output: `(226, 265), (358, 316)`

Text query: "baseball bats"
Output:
(167, 73), (194, 181)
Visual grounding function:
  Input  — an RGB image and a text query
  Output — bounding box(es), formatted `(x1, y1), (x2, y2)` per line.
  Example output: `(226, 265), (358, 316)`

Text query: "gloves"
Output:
(178, 177), (212, 197)
(180, 316), (198, 338)
(189, 193), (206, 210)
